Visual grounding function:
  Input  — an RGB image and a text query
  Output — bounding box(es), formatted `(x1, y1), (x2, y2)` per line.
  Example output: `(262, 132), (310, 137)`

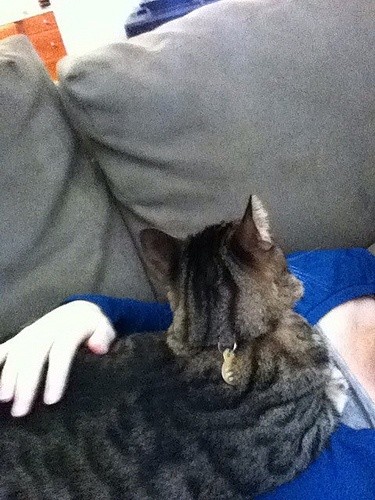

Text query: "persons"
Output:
(0, 245), (374, 500)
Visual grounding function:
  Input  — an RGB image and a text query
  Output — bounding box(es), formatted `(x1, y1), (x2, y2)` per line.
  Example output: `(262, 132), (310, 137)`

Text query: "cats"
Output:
(0, 194), (351, 500)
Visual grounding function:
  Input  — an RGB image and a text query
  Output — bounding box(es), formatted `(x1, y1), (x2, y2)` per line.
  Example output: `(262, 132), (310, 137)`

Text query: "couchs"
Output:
(1, 0), (375, 338)
